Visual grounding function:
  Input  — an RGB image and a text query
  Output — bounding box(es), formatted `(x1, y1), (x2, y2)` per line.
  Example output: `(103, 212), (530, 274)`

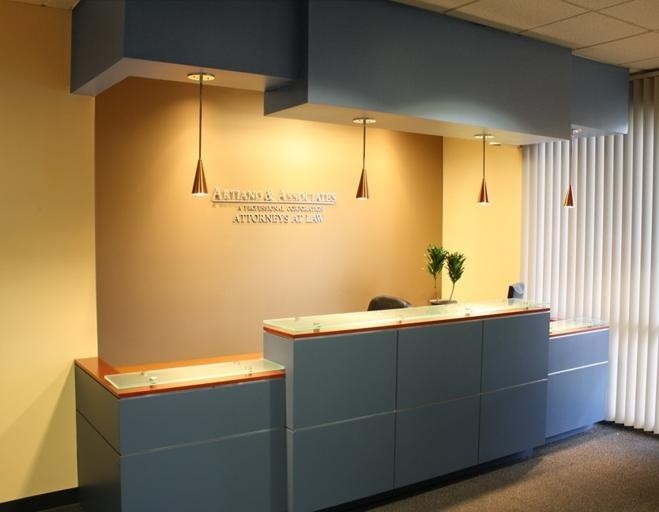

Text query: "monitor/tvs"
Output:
(508, 284), (525, 299)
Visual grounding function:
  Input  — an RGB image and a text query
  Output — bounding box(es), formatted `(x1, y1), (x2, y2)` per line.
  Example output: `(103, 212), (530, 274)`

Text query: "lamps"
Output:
(475, 135), (494, 206)
(190, 73), (213, 196)
(563, 130), (579, 206)
(352, 119), (376, 200)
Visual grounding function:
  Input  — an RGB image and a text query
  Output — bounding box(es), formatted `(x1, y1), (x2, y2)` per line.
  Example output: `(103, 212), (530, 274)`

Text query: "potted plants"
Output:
(425, 242), (466, 305)
